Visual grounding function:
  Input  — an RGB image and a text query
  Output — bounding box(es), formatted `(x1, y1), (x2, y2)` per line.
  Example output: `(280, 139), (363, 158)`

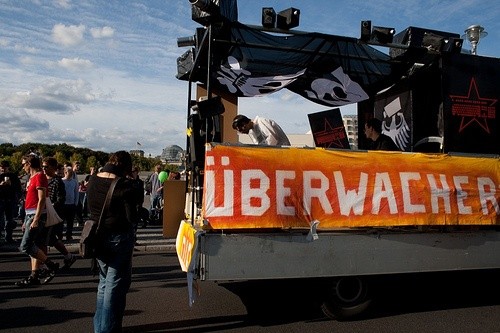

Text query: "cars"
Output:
(136, 176), (152, 229)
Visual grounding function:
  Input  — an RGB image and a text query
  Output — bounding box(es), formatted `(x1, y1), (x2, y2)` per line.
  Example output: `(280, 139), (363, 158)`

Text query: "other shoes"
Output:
(60, 254), (77, 271)
(15, 276), (40, 288)
(6, 240), (17, 244)
(58, 237), (63, 241)
(44, 263), (59, 283)
(66, 237), (73, 241)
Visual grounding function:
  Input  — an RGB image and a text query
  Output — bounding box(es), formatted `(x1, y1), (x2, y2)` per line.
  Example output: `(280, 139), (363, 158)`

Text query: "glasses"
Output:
(363, 127), (369, 132)
(41, 165), (50, 168)
(22, 162), (28, 167)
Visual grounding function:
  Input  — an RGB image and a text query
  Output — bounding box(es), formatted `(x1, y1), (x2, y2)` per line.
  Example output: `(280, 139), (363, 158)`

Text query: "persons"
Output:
(364, 119), (399, 151)
(0, 150), (103, 286)
(131, 164), (181, 227)
(87, 151), (136, 333)
(232, 115), (291, 146)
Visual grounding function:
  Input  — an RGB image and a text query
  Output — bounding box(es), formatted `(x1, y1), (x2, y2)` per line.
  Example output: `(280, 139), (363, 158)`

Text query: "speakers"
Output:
(187, 114), (223, 164)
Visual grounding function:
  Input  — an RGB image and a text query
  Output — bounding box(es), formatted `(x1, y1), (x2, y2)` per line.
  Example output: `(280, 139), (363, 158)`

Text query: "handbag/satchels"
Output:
(79, 219), (101, 259)
(46, 197), (63, 227)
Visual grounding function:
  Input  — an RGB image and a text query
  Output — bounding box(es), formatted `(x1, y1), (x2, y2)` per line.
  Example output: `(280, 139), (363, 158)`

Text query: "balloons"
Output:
(158, 171), (167, 182)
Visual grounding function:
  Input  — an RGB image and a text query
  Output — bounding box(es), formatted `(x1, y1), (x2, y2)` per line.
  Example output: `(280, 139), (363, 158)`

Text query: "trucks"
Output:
(173, 139), (500, 323)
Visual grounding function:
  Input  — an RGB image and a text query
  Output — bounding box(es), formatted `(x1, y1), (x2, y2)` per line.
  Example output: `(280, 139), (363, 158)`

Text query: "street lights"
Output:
(462, 24), (488, 56)
